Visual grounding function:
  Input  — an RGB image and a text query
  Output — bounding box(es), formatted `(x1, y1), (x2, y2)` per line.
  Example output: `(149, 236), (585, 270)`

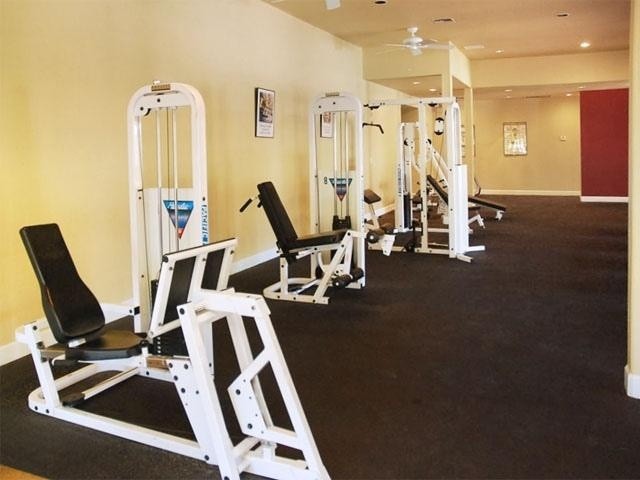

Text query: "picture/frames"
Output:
(255, 87), (275, 138)
(320, 114), (333, 139)
(461, 125), (476, 158)
(503, 122), (528, 156)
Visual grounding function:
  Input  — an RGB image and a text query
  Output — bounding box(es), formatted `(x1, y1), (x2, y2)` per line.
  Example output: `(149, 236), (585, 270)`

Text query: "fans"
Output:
(386, 27), (453, 56)
